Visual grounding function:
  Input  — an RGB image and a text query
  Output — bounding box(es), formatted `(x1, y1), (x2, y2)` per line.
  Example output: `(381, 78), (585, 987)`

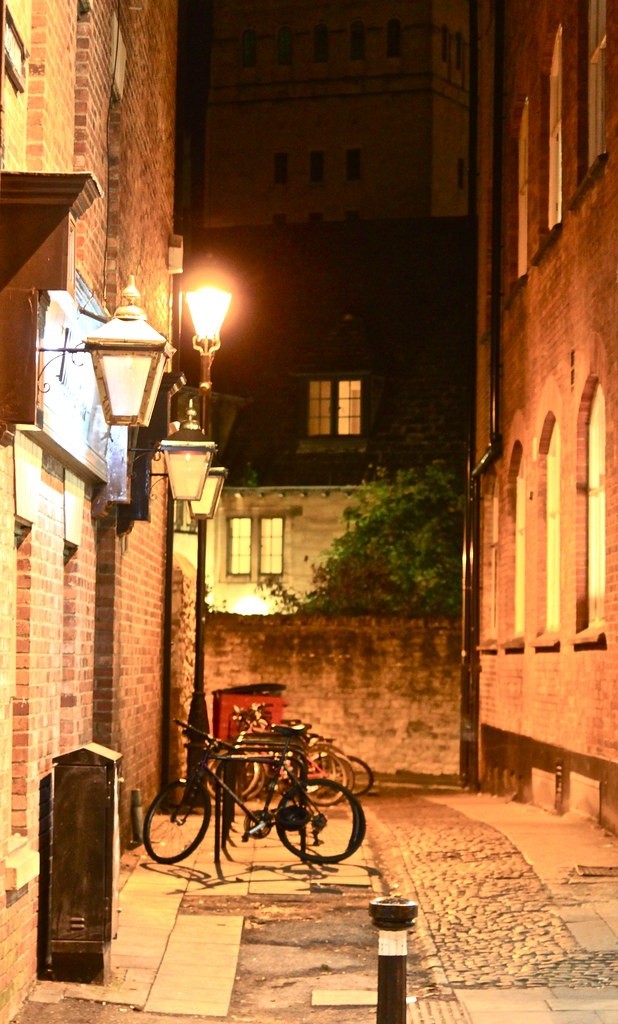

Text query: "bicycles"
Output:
(141, 716), (366, 865)
(204, 702), (373, 807)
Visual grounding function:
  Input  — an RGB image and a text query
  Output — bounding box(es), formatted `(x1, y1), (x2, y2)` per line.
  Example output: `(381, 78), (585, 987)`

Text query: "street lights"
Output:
(185, 285), (234, 434)
(182, 467), (230, 805)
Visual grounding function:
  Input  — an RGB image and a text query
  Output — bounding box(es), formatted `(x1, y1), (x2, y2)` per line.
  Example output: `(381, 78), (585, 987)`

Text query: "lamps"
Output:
(36, 274), (178, 428)
(127, 399), (219, 502)
(149, 443), (229, 520)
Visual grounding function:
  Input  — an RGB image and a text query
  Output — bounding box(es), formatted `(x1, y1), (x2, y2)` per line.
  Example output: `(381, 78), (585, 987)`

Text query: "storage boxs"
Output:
(211, 684), (289, 746)
